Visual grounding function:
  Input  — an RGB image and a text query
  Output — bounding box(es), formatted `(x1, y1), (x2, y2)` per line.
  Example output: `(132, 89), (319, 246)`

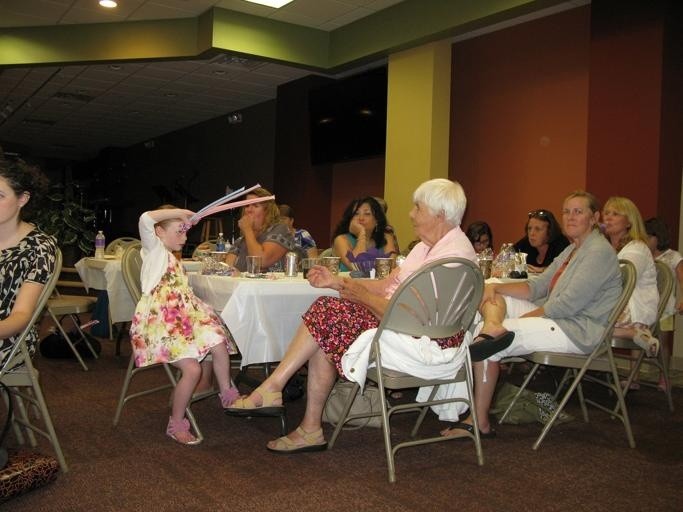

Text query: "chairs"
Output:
(594, 257), (678, 420)
(491, 258), (640, 453)
(111, 241), (235, 441)
(32, 234), (215, 373)
(317, 248), (334, 269)
(0, 242), (69, 481)
(327, 256), (484, 484)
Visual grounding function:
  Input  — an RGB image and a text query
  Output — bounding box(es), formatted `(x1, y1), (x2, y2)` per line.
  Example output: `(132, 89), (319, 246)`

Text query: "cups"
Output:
(322, 256), (341, 275)
(301, 257), (320, 279)
(479, 259), (492, 279)
(373, 257), (393, 281)
(246, 255), (261, 275)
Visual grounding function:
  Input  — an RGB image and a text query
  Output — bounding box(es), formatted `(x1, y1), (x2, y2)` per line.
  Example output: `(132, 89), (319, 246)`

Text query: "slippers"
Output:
(468, 331), (514, 362)
(449, 423), (496, 442)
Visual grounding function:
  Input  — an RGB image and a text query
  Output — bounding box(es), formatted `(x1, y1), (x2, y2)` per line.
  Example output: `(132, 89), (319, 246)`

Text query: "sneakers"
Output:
(621, 380), (640, 390)
(659, 377), (672, 392)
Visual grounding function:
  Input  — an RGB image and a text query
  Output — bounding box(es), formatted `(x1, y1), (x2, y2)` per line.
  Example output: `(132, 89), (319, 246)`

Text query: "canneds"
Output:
(285, 252), (299, 277)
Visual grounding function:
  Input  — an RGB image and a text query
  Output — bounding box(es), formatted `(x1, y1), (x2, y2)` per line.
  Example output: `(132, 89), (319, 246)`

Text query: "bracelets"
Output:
(355, 233), (368, 241)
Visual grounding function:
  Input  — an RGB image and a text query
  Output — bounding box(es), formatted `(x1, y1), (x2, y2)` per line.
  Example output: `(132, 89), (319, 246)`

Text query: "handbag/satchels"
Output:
(322, 377), (392, 431)
(488, 381), (575, 426)
(1, 450), (58, 502)
(39, 313), (101, 362)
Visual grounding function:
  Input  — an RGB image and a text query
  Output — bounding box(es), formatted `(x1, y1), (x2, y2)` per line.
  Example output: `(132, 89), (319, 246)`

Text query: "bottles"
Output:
(94, 231), (105, 260)
(215, 232), (226, 253)
(502, 242), (515, 278)
(283, 251), (298, 277)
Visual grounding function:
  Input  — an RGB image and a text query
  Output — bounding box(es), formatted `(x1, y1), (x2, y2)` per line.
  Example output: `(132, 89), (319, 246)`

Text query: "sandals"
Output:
(267, 424), (327, 454)
(633, 328), (660, 358)
(218, 387), (240, 408)
(167, 415), (202, 446)
(223, 386), (285, 417)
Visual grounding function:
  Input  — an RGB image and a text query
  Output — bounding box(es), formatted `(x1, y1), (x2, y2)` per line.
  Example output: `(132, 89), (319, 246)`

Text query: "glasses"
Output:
(473, 239), (490, 246)
(528, 210), (551, 220)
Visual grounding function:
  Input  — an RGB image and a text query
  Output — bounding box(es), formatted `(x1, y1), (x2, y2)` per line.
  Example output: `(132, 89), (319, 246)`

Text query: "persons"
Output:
(329, 196), (404, 401)
(616, 216), (682, 395)
(506, 208), (574, 387)
(221, 177), (483, 456)
(597, 194), (661, 359)
(0, 150), (60, 473)
(438, 188), (626, 440)
(187, 187), (300, 400)
(129, 203), (244, 445)
(275, 194), (493, 276)
